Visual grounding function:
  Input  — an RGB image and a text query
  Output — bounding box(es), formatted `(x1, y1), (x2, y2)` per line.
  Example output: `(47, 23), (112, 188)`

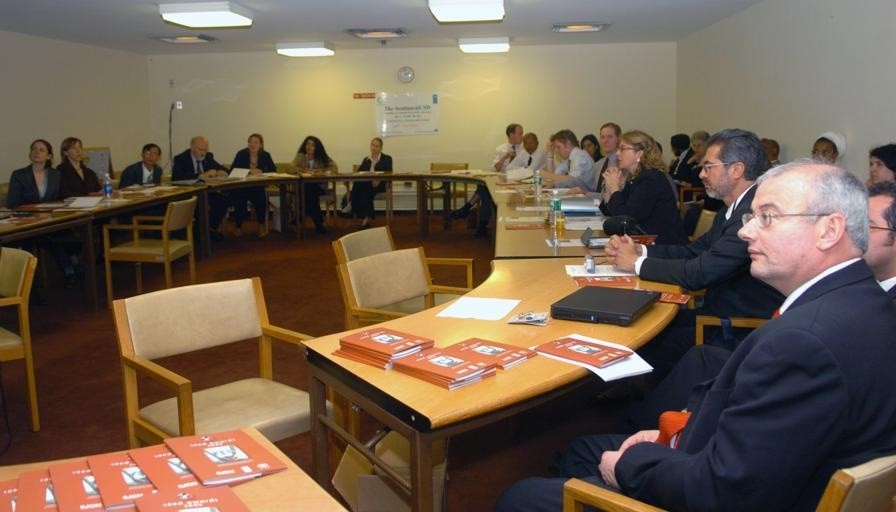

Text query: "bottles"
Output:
(550, 191), (563, 227)
(103, 174), (112, 199)
(535, 170), (542, 195)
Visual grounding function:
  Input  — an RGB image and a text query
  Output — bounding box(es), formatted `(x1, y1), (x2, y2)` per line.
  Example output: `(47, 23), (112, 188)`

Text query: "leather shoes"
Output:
(336, 210), (354, 218)
(257, 225), (268, 237)
(450, 206), (471, 219)
(360, 219), (369, 230)
(207, 229), (224, 244)
(233, 225), (242, 237)
(31, 296), (48, 305)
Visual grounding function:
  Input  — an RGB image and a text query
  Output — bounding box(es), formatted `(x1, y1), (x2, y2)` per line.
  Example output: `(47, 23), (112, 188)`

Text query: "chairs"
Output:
(0, 247), (40, 432)
(428, 163), (469, 213)
(173, 171), (298, 255)
(101, 194), (199, 307)
(333, 227), (474, 312)
(352, 165), (394, 225)
(109, 277), (319, 456)
(338, 247), (434, 328)
(561, 450), (896, 512)
(694, 314), (773, 349)
(321, 161), (337, 223)
(679, 186), (704, 214)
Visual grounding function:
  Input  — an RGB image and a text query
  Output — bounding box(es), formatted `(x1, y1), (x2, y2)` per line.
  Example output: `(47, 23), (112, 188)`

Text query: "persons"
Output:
(287, 137), (338, 234)
(495, 156), (896, 512)
(811, 133), (847, 164)
(678, 131), (706, 201)
(762, 139), (782, 168)
(350, 138), (392, 227)
(506, 133), (547, 171)
(599, 130), (691, 245)
(864, 144), (895, 187)
(605, 129), (787, 371)
(117, 144), (163, 188)
(56, 137), (99, 203)
(540, 130), (595, 181)
(229, 133), (277, 236)
(581, 134), (604, 163)
(7, 139), (61, 208)
(542, 123), (626, 198)
(639, 179), (896, 428)
(172, 136), (228, 231)
(452, 123), (524, 236)
(669, 133), (695, 181)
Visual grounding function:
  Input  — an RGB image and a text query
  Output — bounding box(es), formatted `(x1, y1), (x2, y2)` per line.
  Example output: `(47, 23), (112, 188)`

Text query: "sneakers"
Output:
(60, 255), (95, 279)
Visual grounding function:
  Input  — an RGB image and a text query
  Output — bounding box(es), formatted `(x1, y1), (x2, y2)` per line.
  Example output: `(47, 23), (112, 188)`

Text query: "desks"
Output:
(298, 170), (427, 236)
(496, 201), (614, 260)
(17, 181), (207, 306)
(426, 168), (592, 206)
(296, 256), (681, 512)
(0, 427), (349, 512)
(0, 210), (98, 311)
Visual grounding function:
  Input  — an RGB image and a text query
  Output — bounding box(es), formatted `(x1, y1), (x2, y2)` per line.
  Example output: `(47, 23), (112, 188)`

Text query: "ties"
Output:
(527, 153), (532, 166)
(595, 157), (610, 192)
(654, 305), (785, 448)
(509, 145), (516, 163)
(196, 159), (201, 174)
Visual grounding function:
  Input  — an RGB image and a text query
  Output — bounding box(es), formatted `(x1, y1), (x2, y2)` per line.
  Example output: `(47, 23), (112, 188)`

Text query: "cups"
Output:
(552, 211), (566, 237)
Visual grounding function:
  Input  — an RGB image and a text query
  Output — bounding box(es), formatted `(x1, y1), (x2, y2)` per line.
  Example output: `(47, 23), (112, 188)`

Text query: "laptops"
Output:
(551, 285), (662, 326)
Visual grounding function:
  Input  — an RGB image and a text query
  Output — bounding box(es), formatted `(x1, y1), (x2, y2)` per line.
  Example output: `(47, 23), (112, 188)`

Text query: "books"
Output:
(659, 292), (690, 303)
(1, 429), (286, 511)
(573, 277), (637, 289)
(333, 326), (535, 390)
(533, 335), (634, 366)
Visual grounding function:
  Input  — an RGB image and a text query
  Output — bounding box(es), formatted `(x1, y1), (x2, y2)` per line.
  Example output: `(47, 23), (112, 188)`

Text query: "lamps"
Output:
(458, 37), (509, 53)
(160, 1), (252, 29)
(275, 40), (334, 58)
(427, 0), (506, 23)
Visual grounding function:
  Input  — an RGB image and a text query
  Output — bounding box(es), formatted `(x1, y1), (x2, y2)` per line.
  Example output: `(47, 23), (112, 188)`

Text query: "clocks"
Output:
(397, 65), (412, 83)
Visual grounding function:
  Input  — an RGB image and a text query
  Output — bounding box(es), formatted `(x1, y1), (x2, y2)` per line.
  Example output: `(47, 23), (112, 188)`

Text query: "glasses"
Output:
(867, 222), (892, 233)
(741, 211), (828, 227)
(697, 162), (726, 168)
(620, 146), (635, 151)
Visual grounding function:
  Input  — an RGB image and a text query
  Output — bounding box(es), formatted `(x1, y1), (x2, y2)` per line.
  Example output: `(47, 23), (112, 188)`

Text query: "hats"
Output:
(810, 132), (845, 159)
